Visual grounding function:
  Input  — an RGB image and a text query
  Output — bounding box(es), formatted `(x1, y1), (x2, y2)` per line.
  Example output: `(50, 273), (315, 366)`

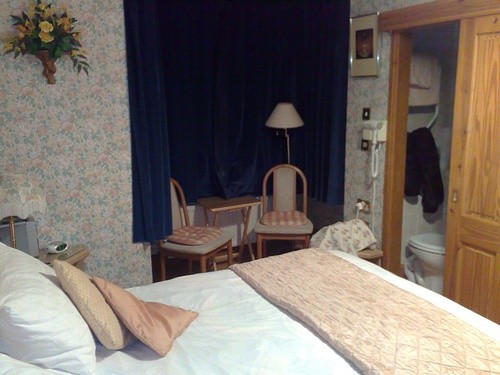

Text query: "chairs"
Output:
(253, 165), (313, 257)
(158, 178), (233, 282)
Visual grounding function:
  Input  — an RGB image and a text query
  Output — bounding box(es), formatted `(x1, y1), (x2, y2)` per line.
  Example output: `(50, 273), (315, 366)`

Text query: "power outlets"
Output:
(355, 197), (371, 215)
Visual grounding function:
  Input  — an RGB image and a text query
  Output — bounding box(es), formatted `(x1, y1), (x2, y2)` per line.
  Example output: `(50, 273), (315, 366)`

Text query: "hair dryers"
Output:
(364, 121), (383, 145)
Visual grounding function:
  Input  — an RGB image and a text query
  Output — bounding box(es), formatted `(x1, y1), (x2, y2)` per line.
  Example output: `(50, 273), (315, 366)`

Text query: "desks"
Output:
(198, 194), (263, 262)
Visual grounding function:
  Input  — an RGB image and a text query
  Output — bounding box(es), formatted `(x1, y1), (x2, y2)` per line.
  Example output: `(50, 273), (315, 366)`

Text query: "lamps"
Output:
(267, 103), (306, 164)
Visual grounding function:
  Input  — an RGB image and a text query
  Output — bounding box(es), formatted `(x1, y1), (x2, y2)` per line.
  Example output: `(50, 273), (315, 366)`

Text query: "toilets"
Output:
(408, 232), (446, 296)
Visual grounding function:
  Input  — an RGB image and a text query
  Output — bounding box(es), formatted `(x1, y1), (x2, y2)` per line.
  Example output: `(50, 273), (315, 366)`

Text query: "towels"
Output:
(410, 53), (433, 88)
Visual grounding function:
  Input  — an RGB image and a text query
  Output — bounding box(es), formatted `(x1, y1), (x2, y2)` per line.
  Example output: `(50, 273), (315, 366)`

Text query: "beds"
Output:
(1, 249), (500, 375)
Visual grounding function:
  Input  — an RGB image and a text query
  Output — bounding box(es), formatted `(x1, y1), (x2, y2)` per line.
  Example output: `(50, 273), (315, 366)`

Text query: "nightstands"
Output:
(31, 242), (89, 278)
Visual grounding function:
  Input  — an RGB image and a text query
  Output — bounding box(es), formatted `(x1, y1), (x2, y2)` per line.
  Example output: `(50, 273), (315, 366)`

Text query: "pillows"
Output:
(91, 276), (199, 358)
(51, 260), (142, 351)
(0, 241), (96, 374)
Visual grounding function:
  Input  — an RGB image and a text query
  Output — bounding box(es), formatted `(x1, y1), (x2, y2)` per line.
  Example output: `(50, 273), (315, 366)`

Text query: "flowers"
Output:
(0, 0), (91, 84)
